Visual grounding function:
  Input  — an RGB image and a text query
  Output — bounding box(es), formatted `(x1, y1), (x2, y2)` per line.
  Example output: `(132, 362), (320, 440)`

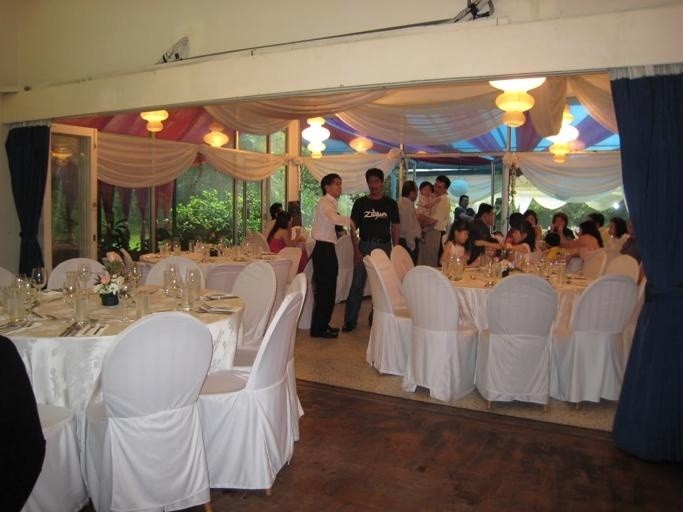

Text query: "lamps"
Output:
(138, 108), (168, 134)
(544, 108), (579, 162)
(482, 76), (544, 130)
(348, 130), (374, 155)
(302, 115), (330, 160)
(204, 122), (228, 150)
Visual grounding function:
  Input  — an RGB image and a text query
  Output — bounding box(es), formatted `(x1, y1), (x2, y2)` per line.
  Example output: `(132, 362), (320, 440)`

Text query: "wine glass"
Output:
(293, 224), (312, 243)
(2, 264), (240, 338)
(538, 233), (617, 249)
(435, 250), (589, 291)
(145, 235), (278, 262)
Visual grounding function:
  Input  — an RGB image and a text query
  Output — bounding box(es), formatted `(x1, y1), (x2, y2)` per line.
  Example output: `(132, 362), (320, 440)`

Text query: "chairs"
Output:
(295, 215), (647, 413)
(2, 214), (308, 511)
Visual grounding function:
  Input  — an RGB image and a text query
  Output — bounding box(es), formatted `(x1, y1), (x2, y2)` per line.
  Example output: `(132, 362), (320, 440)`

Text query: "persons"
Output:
(0, 333), (46, 511)
(309, 173), (355, 339)
(342, 169), (401, 332)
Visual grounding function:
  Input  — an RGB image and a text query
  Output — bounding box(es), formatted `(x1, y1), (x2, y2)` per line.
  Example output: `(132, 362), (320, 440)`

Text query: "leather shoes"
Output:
(309, 324), (340, 338)
(340, 321), (357, 332)
